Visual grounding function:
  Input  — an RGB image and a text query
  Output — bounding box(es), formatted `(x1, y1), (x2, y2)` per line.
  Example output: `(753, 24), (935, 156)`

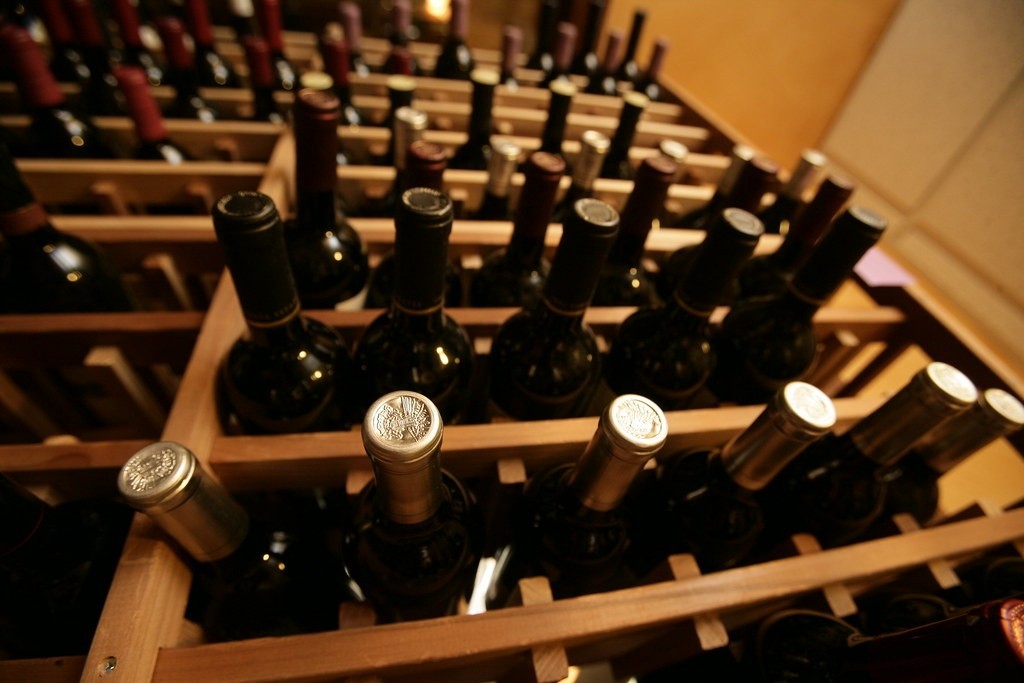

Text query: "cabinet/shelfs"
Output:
(2, 0), (1023, 680)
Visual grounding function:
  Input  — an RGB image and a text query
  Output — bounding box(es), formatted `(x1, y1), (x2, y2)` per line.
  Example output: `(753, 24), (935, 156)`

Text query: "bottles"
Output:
(0, 1), (1024, 683)
